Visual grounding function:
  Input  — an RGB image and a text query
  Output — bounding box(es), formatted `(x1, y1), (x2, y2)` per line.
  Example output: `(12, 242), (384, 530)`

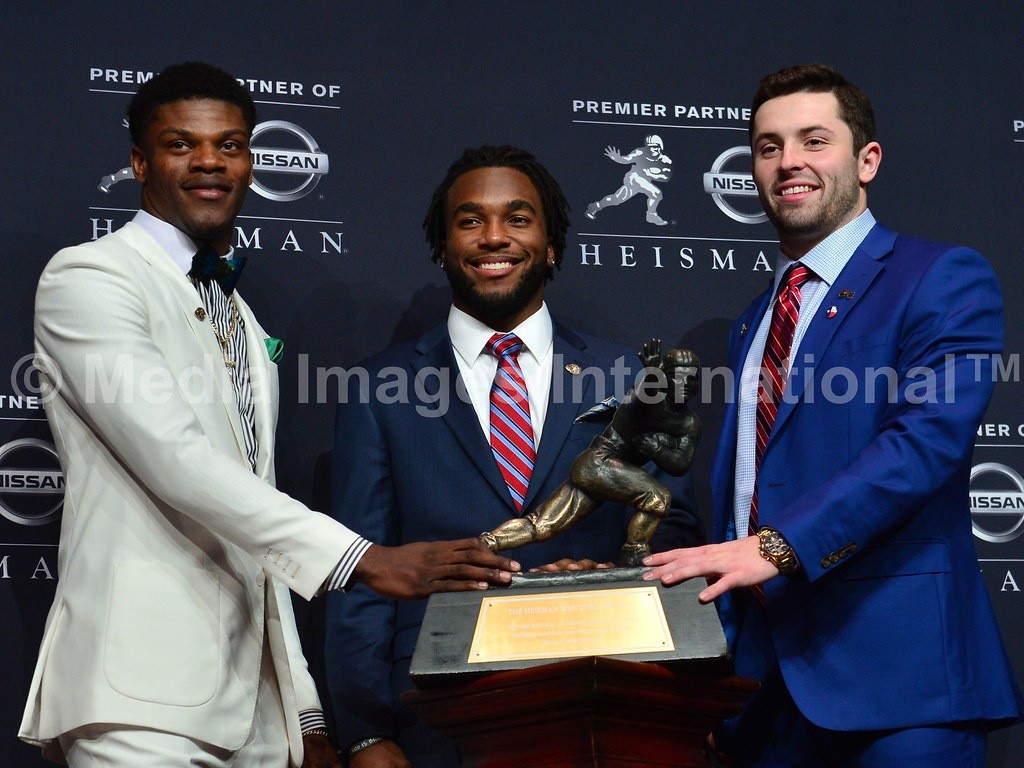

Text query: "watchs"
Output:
(757, 528), (796, 576)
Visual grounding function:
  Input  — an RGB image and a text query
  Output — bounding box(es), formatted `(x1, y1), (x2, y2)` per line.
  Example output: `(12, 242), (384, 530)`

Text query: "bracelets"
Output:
(349, 736), (383, 754)
(301, 729), (329, 737)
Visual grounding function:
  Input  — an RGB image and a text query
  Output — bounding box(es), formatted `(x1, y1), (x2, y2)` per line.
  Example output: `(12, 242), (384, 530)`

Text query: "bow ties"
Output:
(189, 247), (237, 299)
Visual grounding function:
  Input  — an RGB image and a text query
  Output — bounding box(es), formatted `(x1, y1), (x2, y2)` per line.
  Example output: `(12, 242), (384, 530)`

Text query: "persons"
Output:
(479, 336), (700, 557)
(314, 135), (713, 768)
(14, 61), (525, 768)
(658, 63), (1024, 768)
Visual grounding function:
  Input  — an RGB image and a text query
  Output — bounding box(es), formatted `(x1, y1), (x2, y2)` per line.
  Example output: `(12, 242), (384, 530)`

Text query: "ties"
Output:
(748, 265), (815, 598)
(480, 333), (537, 514)
